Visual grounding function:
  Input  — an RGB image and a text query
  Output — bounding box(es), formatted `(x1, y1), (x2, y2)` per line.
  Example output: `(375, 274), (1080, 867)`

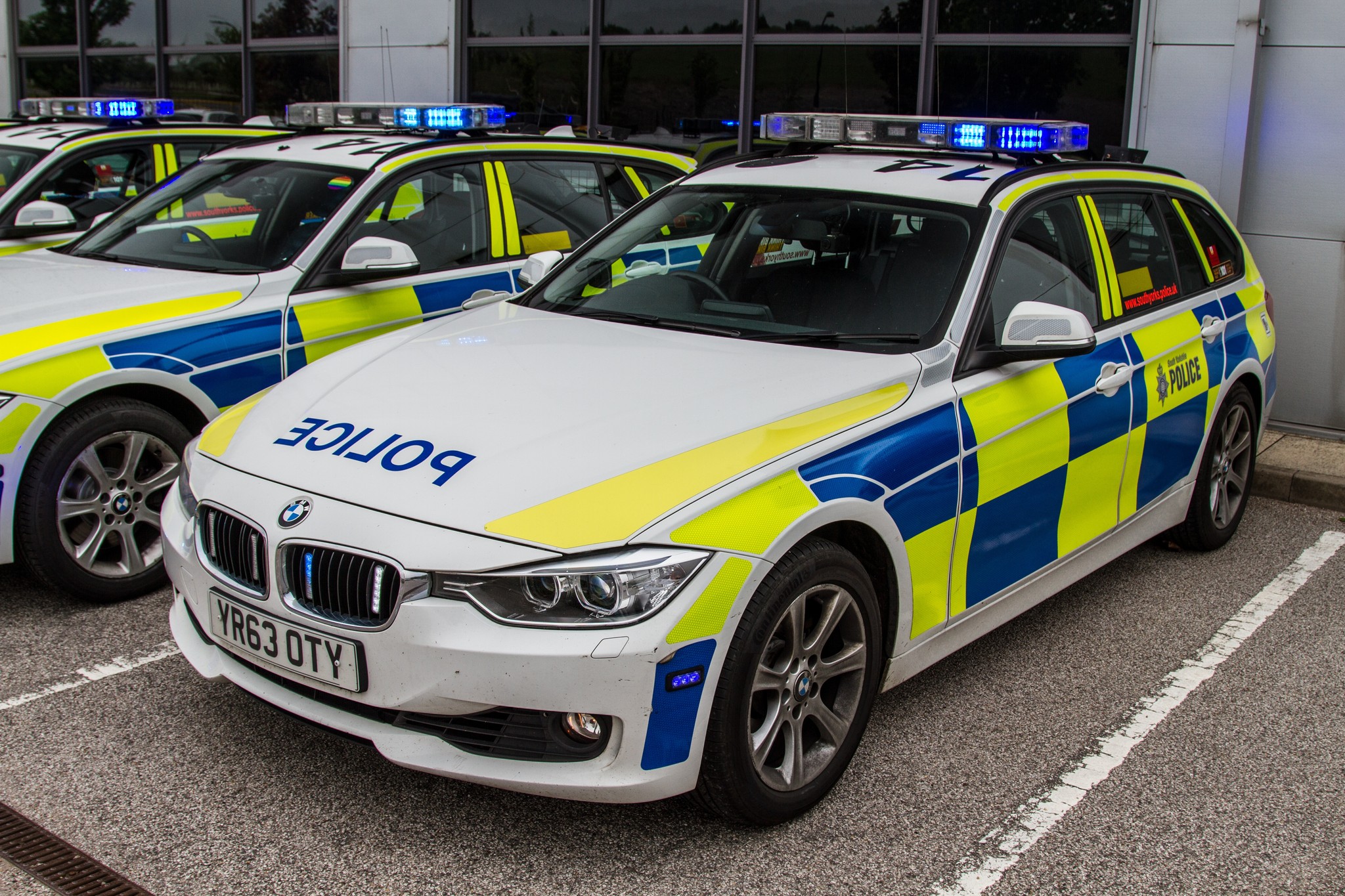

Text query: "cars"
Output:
(0, 100), (694, 606)
(157, 105), (1284, 829)
(2, 103), (292, 258)
(156, 108), (240, 124)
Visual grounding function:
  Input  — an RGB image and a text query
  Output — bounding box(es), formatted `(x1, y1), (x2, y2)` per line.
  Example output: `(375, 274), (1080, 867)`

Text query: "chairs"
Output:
(44, 159), (97, 207)
(266, 184), (347, 271)
(755, 219), (858, 327)
